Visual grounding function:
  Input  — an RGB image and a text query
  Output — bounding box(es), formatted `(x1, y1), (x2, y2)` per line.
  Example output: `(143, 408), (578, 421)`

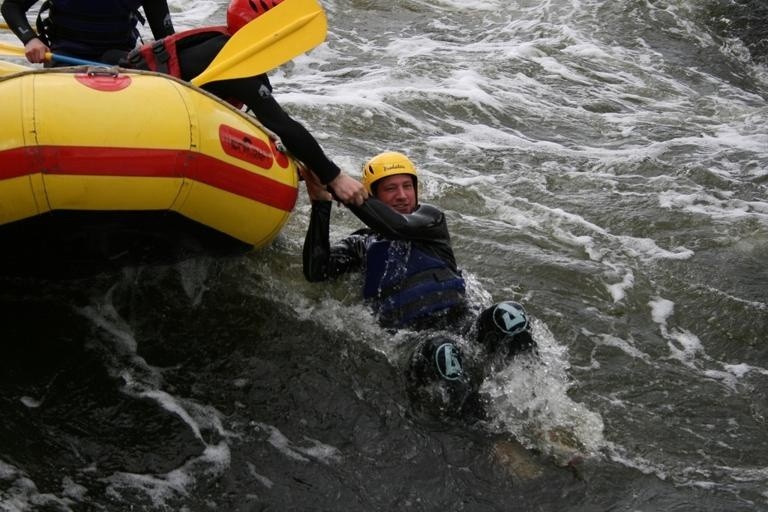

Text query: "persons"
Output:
(0, 0), (175, 68)
(303, 150), (538, 426)
(119, 0), (369, 206)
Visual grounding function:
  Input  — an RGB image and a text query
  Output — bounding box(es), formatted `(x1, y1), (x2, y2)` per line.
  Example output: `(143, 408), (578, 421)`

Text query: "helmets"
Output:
(227, 1), (281, 36)
(361, 152), (418, 207)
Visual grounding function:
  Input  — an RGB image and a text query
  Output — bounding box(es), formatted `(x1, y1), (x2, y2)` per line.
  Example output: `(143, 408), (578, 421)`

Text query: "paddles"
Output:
(187, 0), (327, 87)
(0, 61), (30, 74)
(0, 40), (113, 68)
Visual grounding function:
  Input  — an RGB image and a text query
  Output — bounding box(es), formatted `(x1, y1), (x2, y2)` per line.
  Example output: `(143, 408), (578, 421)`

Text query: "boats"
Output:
(0, 54), (298, 251)
